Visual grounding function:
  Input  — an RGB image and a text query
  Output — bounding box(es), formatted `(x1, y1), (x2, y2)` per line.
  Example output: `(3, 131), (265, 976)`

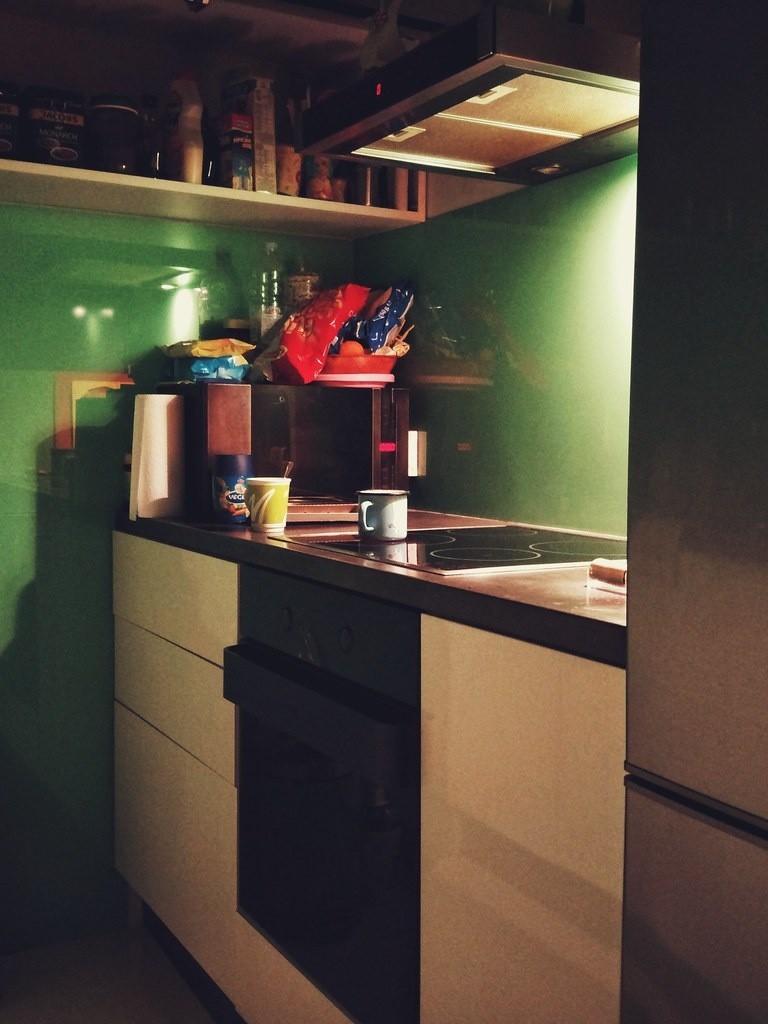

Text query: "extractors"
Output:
(292, 0), (640, 185)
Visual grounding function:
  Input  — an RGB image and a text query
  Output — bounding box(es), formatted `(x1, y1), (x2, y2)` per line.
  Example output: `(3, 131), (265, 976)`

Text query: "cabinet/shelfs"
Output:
(105, 537), (626, 1024)
(0, 0), (426, 239)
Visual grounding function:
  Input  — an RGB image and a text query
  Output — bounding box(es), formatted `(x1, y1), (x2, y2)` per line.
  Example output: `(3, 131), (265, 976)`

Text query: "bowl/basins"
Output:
(316, 354), (399, 388)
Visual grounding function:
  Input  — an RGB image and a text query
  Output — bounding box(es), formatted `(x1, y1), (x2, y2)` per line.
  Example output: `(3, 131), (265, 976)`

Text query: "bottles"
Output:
(200, 103), (224, 184)
(144, 93), (163, 175)
(261, 242), (285, 336)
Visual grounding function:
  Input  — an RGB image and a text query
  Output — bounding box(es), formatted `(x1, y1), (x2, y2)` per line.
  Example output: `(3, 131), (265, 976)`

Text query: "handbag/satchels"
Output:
(267, 283), (371, 386)
(328, 285), (420, 355)
(162, 338), (258, 383)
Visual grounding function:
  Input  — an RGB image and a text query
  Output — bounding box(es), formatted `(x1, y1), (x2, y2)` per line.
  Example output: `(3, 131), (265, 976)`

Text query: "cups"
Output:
(289, 272), (321, 312)
(222, 318), (255, 343)
(121, 452), (133, 511)
(87, 95), (143, 174)
(356, 489), (410, 541)
(210, 454), (253, 525)
(246, 477), (292, 532)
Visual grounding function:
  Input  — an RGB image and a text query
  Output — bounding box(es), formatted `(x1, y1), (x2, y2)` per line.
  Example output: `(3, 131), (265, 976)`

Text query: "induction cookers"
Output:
(307, 524), (627, 575)
